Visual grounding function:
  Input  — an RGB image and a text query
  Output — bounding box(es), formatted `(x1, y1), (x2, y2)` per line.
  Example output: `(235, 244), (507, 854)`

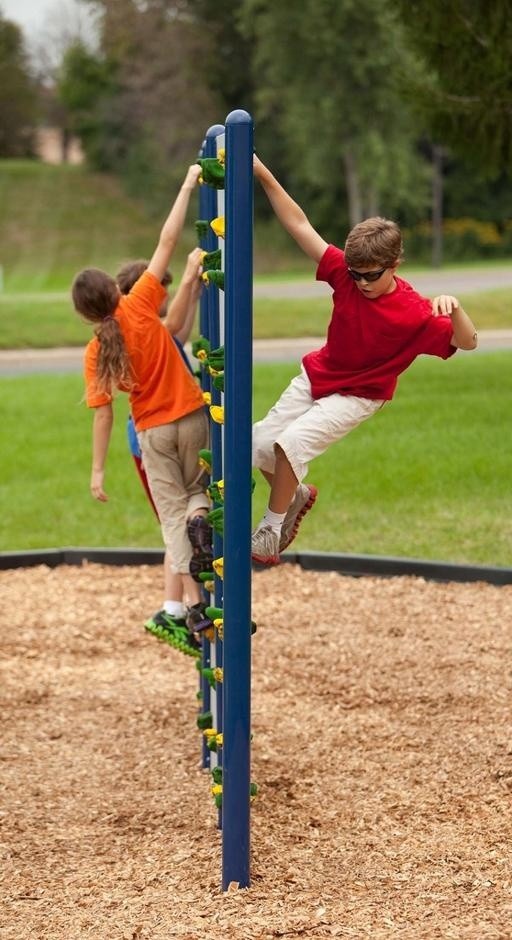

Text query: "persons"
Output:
(250, 151), (478, 569)
(70, 164), (212, 635)
(116, 248), (204, 657)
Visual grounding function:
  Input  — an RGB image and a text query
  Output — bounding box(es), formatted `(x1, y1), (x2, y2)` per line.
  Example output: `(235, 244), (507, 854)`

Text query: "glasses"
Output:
(347, 267), (385, 281)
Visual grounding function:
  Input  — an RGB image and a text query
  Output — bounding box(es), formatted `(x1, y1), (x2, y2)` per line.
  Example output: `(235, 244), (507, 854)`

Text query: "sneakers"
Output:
(143, 602), (213, 657)
(251, 483), (318, 565)
(188, 515), (214, 583)
